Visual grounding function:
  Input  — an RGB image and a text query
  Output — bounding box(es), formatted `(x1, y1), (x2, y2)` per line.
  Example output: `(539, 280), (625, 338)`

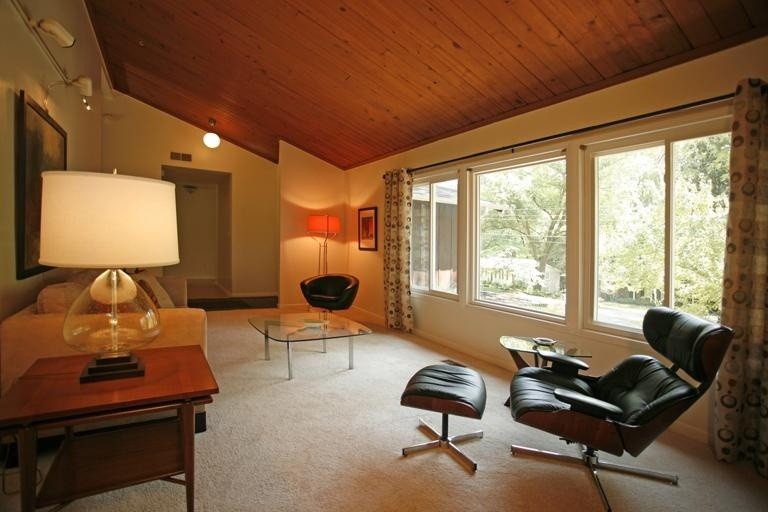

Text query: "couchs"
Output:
(1, 272), (207, 445)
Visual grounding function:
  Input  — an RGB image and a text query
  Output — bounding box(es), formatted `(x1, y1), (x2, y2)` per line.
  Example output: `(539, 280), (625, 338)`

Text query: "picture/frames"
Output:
(18, 90), (68, 280)
(357, 207), (378, 251)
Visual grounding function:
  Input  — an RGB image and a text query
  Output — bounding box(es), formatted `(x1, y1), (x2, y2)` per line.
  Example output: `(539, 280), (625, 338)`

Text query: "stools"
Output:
(400, 364), (486, 472)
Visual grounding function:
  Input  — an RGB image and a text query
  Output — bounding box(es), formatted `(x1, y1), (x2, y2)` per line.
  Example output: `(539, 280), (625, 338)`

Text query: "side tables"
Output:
(500, 334), (592, 406)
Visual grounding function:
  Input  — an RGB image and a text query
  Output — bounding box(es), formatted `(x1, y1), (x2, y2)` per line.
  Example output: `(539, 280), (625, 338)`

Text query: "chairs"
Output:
(510, 307), (735, 512)
(300, 274), (356, 313)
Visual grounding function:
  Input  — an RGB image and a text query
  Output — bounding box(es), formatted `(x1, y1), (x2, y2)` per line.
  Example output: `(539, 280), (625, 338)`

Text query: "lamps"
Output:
(31, 17), (94, 99)
(308, 214), (341, 274)
(38, 170), (178, 385)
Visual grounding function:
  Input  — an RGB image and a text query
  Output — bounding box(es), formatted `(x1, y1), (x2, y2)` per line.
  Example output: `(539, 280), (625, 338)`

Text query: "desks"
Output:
(2, 345), (219, 512)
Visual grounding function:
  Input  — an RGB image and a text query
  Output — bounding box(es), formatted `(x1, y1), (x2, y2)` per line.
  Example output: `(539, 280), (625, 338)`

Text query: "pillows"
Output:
(97, 269), (176, 313)
